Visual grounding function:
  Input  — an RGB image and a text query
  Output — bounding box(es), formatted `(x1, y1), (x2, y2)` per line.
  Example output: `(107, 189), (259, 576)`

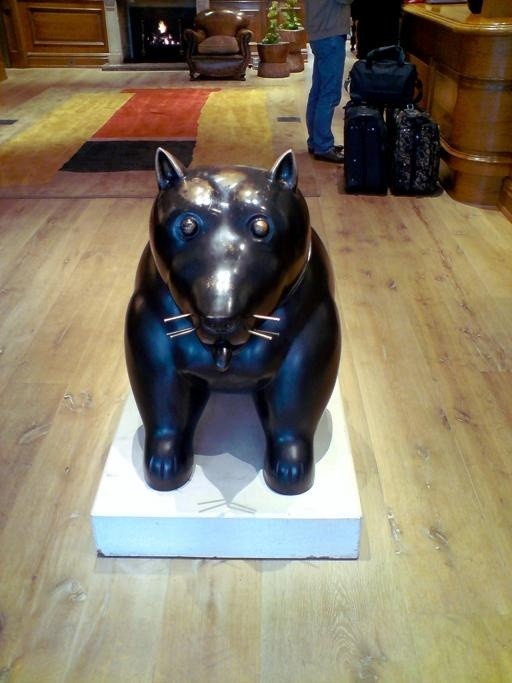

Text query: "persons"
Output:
(304, 0), (351, 164)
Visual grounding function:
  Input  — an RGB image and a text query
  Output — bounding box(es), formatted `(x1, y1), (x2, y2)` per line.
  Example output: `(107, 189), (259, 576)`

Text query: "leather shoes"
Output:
(307, 143), (344, 155)
(314, 150), (345, 164)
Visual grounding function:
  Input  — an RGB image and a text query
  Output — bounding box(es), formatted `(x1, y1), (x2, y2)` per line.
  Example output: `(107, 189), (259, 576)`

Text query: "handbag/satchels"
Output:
(345, 43), (424, 108)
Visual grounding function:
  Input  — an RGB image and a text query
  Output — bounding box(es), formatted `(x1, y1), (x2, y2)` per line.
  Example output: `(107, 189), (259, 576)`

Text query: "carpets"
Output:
(0, 85), (321, 198)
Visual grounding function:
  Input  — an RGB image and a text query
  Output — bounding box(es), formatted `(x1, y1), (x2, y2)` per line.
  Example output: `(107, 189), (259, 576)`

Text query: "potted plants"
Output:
(257, 33), (290, 78)
(268, 0), (305, 73)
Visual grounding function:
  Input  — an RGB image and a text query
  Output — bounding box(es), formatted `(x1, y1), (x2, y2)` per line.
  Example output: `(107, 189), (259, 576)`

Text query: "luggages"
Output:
(390, 104), (440, 196)
(343, 100), (390, 196)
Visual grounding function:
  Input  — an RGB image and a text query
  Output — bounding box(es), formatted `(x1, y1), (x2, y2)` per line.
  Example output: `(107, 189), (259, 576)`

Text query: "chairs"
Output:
(184, 8), (254, 81)
